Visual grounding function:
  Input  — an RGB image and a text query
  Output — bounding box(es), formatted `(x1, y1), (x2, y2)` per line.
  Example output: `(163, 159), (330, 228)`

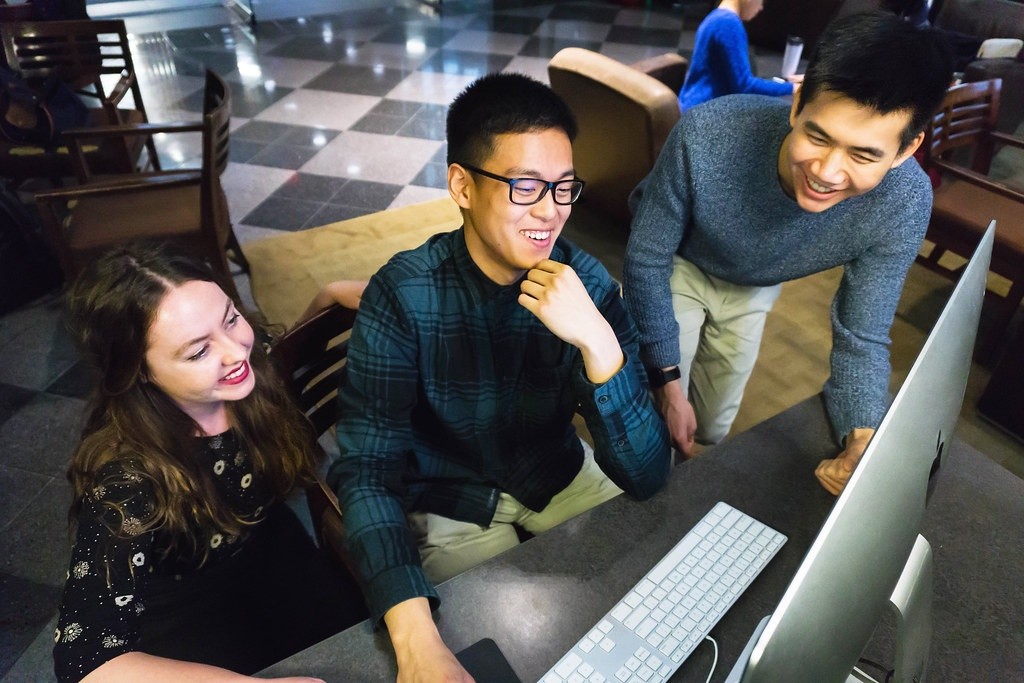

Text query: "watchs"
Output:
(647, 366), (682, 391)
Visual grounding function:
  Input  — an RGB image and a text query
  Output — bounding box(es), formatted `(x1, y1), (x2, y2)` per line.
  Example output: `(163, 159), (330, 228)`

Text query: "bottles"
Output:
(781, 35), (805, 78)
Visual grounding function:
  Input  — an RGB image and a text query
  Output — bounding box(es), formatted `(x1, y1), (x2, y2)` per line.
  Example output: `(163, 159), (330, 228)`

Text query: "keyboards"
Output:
(536, 501), (787, 683)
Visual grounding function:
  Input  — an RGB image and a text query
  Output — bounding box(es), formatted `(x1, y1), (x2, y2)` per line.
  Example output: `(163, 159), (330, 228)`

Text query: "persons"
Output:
(50, 236), (368, 683)
(677, 0), (806, 118)
(623, 9), (949, 500)
(324, 71), (673, 683)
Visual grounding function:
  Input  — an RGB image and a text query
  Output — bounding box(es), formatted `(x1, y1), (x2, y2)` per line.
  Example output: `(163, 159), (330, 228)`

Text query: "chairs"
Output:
(0, 19), (161, 218)
(34, 68), (252, 311)
(925, 78), (1024, 356)
(267, 304), (364, 596)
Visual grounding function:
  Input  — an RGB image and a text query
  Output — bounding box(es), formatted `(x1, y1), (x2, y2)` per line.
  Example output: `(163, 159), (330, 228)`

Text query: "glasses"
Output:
(457, 162), (586, 206)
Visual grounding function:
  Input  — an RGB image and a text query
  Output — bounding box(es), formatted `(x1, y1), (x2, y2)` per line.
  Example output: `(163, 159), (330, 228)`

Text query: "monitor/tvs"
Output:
(724, 220), (997, 683)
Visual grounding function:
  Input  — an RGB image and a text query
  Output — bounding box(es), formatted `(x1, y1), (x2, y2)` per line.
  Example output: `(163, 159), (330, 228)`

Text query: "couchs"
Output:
(916, 0), (1024, 161)
(547, 48), (694, 229)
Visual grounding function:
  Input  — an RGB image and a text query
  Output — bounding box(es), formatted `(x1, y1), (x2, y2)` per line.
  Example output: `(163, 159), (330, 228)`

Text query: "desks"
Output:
(252, 389), (1024, 683)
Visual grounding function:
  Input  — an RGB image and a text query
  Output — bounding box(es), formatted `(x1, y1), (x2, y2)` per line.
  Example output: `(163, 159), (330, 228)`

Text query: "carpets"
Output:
(232, 195), (1023, 480)
